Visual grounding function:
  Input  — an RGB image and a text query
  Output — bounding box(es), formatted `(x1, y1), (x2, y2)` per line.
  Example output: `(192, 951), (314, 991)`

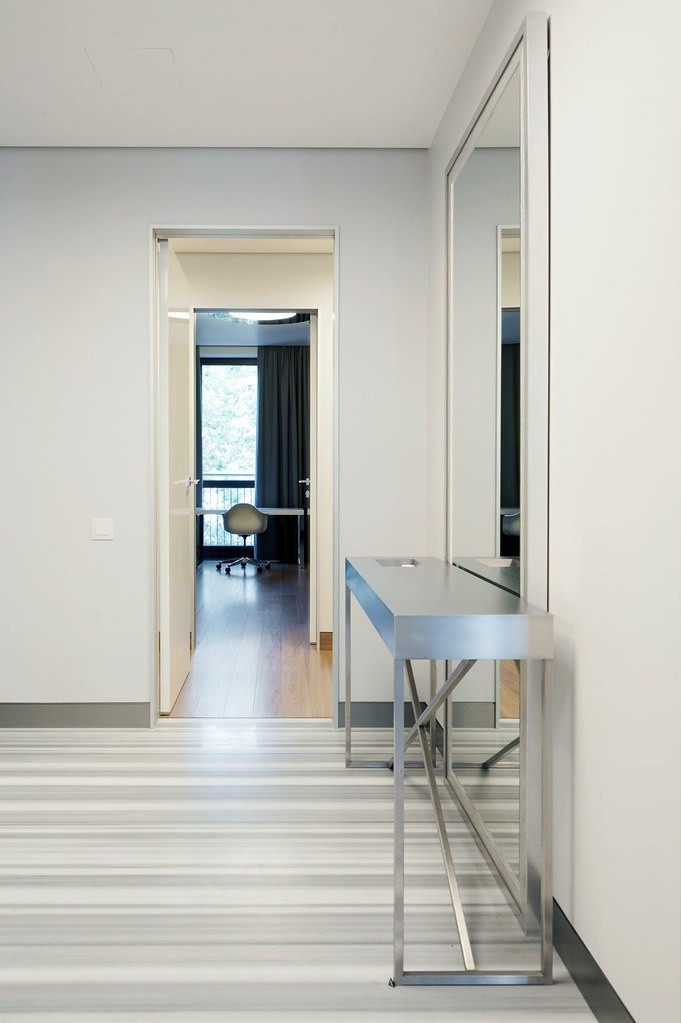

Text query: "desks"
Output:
(196, 508), (304, 570)
(345, 552), (554, 985)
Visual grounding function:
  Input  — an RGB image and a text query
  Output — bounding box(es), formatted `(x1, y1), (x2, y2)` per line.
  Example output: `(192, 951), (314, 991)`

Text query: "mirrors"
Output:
(432, 9), (548, 938)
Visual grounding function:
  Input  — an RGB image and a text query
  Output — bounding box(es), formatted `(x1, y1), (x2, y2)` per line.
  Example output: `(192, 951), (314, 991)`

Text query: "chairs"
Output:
(216, 504), (271, 573)
